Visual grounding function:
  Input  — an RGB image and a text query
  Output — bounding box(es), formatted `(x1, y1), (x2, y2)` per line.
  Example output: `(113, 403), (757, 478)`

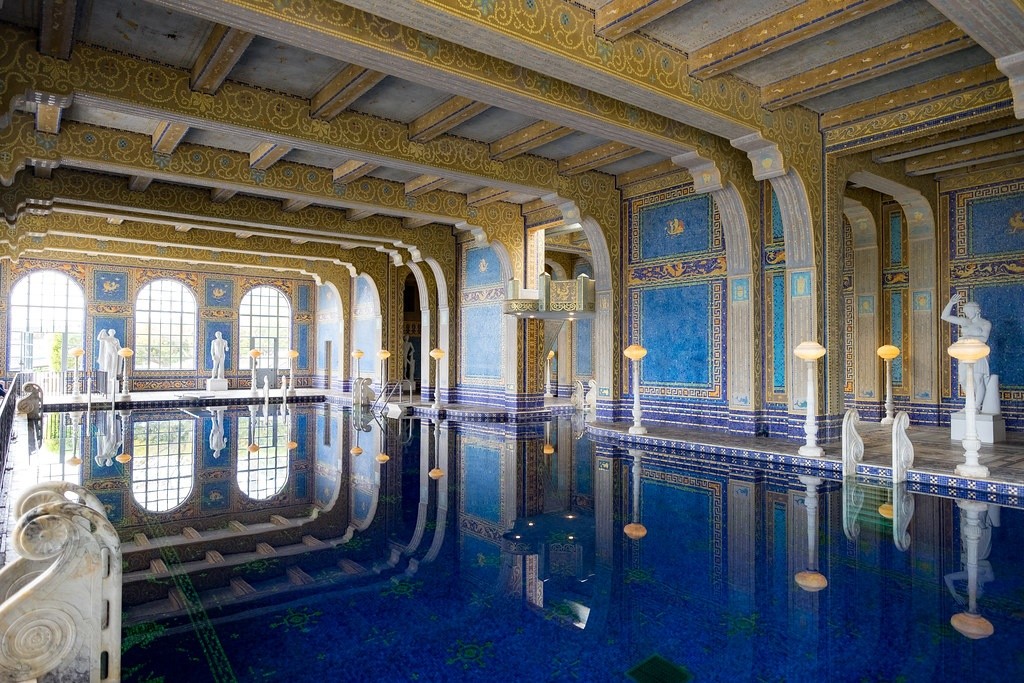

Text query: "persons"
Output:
(941, 294), (992, 414)
(209, 417), (227, 459)
(96, 419), (122, 466)
(944, 520), (995, 607)
(97, 328), (123, 380)
(211, 331), (229, 379)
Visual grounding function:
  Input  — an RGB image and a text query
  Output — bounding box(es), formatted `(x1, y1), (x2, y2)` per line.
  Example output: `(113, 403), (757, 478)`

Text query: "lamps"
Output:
(375, 453), (390, 467)
(66, 440), (363, 466)
(547, 351), (555, 360)
(284, 350), (299, 360)
(74, 348), (85, 357)
(948, 339), (990, 361)
(794, 567), (830, 591)
(426, 467), (446, 479)
(429, 348), (444, 359)
(249, 350), (262, 358)
(118, 348), (134, 357)
(351, 348), (364, 359)
(377, 350), (391, 361)
(541, 442), (558, 457)
(621, 524), (648, 541)
(877, 345), (901, 359)
(793, 341), (826, 359)
(874, 503), (994, 642)
(623, 344), (648, 360)
